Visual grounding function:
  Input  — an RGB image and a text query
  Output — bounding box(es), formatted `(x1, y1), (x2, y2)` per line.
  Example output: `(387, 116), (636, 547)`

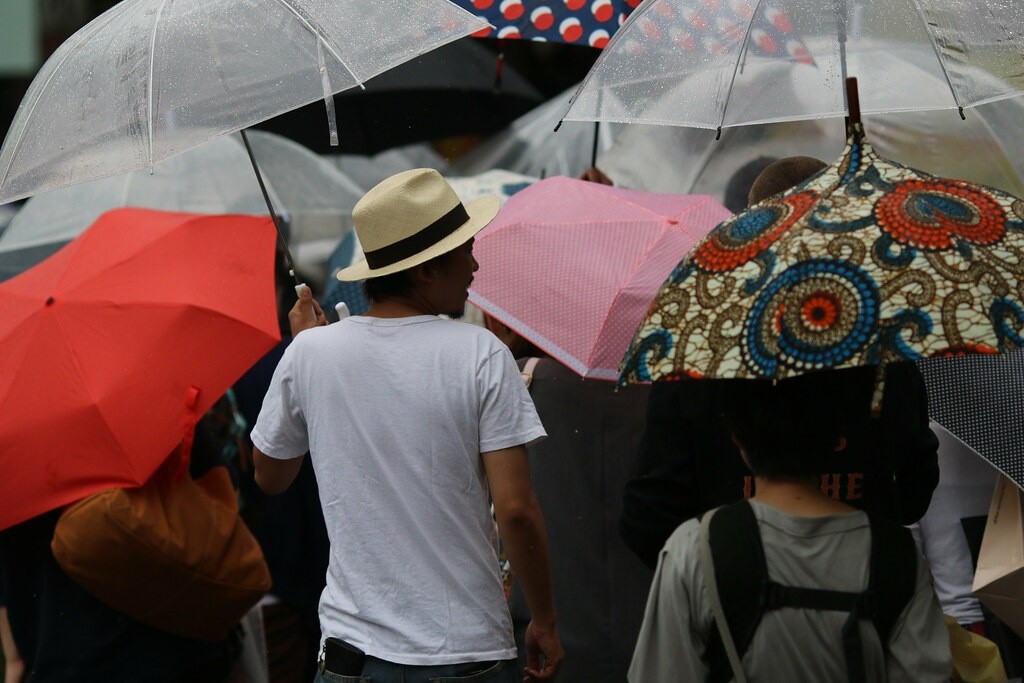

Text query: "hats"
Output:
(336, 168), (500, 280)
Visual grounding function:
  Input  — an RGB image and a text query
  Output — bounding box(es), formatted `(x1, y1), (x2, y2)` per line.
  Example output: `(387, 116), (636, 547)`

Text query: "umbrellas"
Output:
(326, 0), (1024, 386)
(0, 125), (365, 254)
(551, 0), (1024, 155)
(0, 0), (497, 302)
(0, 207), (285, 530)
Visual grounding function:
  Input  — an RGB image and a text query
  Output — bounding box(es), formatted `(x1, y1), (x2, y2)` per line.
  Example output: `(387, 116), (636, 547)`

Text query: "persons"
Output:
(0, 218), (330, 683)
(250, 168), (562, 683)
(488, 157), (1024, 683)
